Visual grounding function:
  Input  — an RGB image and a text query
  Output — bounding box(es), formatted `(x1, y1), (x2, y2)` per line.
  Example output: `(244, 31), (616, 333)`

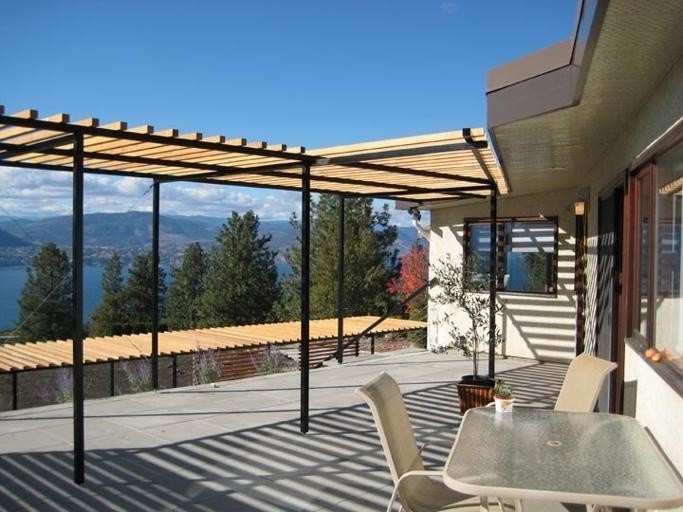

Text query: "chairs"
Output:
(515, 352), (618, 512)
(356, 372), (516, 511)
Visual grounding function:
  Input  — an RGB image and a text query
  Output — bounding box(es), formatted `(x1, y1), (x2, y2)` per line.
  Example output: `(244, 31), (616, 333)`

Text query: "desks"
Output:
(441, 406), (683, 511)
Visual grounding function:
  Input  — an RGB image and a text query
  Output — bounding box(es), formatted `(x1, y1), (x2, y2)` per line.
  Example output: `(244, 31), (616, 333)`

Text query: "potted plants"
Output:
(490, 378), (515, 413)
(422, 252), (510, 417)
